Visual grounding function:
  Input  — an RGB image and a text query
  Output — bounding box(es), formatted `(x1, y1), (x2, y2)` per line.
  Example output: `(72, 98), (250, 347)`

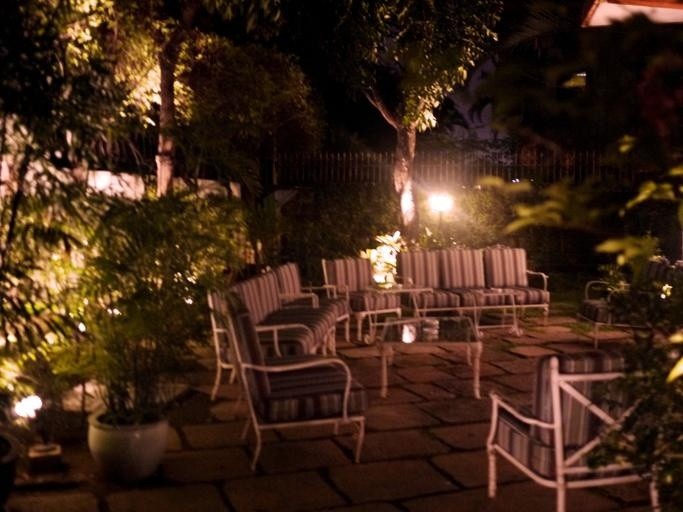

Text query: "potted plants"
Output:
(0, 1), (197, 511)
(52, 193), (246, 479)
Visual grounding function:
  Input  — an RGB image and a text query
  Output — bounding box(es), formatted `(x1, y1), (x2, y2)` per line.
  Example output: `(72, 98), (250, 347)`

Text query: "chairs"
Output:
(278, 264), (352, 348)
(574, 279), (659, 349)
(485, 246), (550, 326)
(397, 247), (480, 342)
(487, 346), (666, 512)
(208, 271), (337, 403)
(443, 246), (517, 329)
(220, 292), (368, 469)
(323, 258), (401, 340)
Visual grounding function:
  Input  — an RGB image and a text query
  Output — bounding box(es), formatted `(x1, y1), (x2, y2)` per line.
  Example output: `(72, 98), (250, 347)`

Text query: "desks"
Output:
(377, 314), (484, 400)
